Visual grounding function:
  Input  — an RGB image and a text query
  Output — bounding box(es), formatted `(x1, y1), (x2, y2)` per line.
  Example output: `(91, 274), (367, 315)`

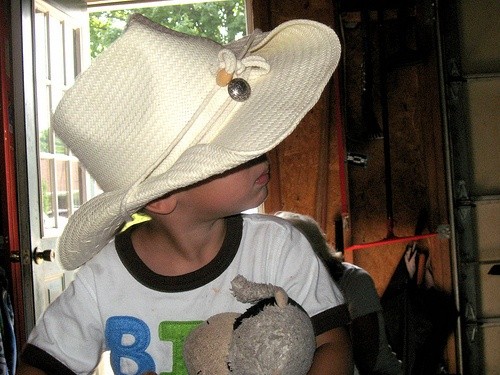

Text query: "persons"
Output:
(17, 12), (357, 375)
(268, 210), (409, 374)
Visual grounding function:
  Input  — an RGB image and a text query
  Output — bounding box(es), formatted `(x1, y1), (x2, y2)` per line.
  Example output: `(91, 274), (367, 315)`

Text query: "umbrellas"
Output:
(381, 252), (458, 375)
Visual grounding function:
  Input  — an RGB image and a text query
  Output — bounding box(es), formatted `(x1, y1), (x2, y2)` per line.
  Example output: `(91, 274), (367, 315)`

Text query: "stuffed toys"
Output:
(185, 274), (317, 374)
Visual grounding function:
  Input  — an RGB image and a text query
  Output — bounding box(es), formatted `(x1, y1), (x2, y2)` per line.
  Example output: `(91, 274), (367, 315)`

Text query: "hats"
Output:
(52, 14), (342, 269)
